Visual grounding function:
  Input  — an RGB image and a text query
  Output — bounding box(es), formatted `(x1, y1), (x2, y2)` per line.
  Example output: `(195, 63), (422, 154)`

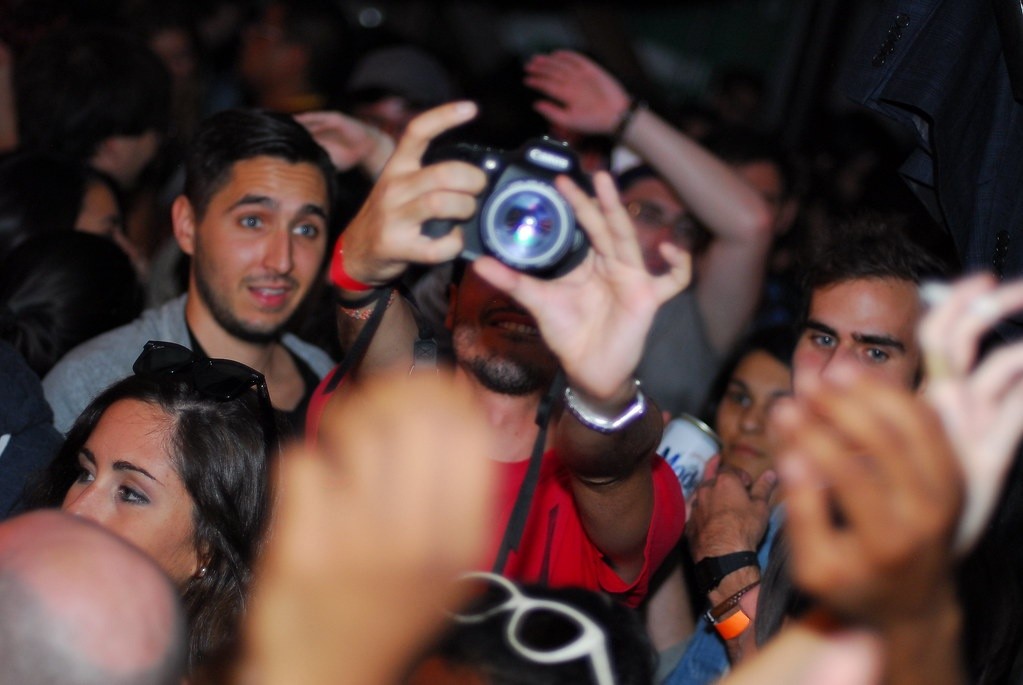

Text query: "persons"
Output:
(385, 569), (658, 685)
(284, 100), (685, 685)
(41, 103), (333, 427)
(698, 152), (1023, 685)
(525, 48), (768, 416)
(0, 0), (345, 340)
(0, 340), (280, 685)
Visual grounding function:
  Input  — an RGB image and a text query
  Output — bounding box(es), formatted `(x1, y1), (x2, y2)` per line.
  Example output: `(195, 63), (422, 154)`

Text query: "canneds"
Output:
(655, 411), (721, 500)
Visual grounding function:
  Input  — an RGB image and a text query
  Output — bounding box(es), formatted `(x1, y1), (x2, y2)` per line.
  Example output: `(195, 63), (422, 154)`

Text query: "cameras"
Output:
(420, 136), (591, 279)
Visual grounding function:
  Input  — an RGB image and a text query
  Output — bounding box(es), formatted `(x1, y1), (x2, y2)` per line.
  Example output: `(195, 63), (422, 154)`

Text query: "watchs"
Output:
(695, 550), (761, 592)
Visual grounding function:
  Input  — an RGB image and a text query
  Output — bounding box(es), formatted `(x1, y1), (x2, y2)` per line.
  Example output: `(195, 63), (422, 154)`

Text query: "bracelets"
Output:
(331, 234), (376, 292)
(703, 580), (766, 641)
(614, 95), (639, 140)
(560, 379), (646, 436)
(338, 288), (399, 318)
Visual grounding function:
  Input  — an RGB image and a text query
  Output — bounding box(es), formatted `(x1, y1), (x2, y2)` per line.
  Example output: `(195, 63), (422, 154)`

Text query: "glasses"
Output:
(440, 571), (615, 685)
(134, 340), (271, 404)
